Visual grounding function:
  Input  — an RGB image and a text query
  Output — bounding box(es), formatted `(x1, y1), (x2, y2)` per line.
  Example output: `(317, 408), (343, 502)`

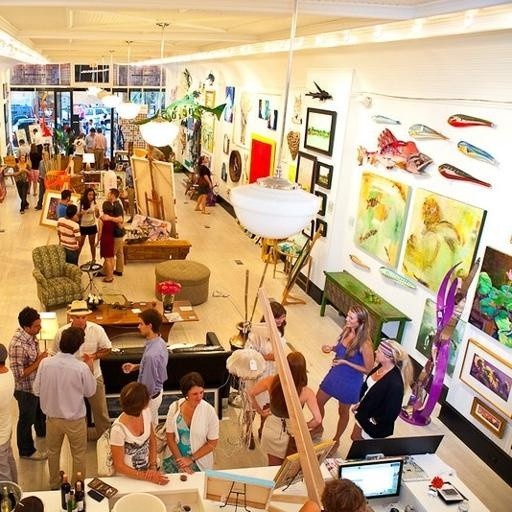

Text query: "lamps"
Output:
(230, 0), (322, 242)
(86, 22), (179, 147)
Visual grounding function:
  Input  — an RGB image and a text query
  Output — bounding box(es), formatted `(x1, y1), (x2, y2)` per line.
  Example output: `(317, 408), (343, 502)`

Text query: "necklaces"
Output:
(376, 368), (386, 375)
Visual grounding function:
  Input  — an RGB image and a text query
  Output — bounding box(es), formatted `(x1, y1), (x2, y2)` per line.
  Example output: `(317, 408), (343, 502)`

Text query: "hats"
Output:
(67, 300), (93, 316)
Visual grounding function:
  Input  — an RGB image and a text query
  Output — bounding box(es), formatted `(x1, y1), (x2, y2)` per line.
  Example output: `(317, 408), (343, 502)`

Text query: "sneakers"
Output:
(20, 203), (42, 214)
(93, 271), (105, 277)
(20, 449), (48, 460)
(113, 271), (122, 276)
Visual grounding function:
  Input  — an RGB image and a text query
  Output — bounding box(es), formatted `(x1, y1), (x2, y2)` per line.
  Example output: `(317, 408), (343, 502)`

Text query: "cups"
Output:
(110, 493), (166, 512)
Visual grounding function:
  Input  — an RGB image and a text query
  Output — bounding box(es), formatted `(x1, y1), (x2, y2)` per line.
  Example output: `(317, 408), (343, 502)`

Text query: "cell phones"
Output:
(87, 489), (105, 503)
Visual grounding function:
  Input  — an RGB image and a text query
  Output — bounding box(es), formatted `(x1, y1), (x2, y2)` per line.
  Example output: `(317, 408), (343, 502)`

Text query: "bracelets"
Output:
(258, 410), (263, 414)
(190, 455), (197, 463)
(136, 470), (139, 479)
(147, 462), (157, 468)
(351, 362), (355, 368)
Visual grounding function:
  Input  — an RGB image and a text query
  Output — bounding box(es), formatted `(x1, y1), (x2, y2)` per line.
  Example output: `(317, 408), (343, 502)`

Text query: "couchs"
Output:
(100, 332), (234, 409)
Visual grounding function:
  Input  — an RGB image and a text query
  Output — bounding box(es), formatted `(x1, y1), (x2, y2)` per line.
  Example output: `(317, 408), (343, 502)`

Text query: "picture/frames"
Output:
(295, 107), (337, 241)
(273, 441), (337, 487)
(204, 469), (275, 511)
(458, 338), (512, 439)
(39, 189), (81, 231)
(87, 385), (222, 428)
(205, 91), (215, 153)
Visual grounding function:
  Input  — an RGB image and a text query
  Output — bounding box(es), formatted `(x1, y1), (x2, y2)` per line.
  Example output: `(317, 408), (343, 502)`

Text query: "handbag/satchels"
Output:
(196, 177), (209, 185)
(153, 422), (167, 453)
(96, 427), (116, 476)
(115, 227), (122, 236)
(209, 194), (217, 206)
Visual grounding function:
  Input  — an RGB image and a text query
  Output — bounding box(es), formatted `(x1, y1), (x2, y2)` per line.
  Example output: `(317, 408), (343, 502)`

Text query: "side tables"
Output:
(80, 264), (103, 295)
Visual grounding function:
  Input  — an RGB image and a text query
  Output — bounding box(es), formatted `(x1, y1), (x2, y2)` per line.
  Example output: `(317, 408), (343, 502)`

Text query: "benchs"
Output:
(124, 240), (192, 264)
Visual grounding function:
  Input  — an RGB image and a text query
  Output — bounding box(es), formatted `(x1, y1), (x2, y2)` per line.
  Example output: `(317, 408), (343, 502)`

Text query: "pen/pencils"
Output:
(45, 340), (46, 350)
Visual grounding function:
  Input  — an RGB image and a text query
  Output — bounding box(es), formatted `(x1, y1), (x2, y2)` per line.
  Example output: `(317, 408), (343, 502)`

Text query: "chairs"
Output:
(32, 245), (84, 312)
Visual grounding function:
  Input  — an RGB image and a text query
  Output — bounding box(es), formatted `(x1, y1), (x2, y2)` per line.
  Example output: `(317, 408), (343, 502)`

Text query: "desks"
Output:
(272, 242), (312, 293)
(320, 269), (413, 360)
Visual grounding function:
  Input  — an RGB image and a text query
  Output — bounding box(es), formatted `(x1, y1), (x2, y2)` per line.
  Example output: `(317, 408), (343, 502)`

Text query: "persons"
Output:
(14, 104), (139, 283)
(121, 308), (169, 427)
(350, 339), (413, 441)
(10, 307), (48, 459)
(322, 478), (368, 512)
(53, 301), (111, 434)
(147, 144), (165, 161)
(193, 155), (214, 213)
(0, 343), (21, 488)
(249, 351), (321, 467)
(244, 300), (287, 442)
(33, 327), (97, 490)
(110, 380), (169, 486)
(161, 372), (219, 474)
(311, 303), (374, 458)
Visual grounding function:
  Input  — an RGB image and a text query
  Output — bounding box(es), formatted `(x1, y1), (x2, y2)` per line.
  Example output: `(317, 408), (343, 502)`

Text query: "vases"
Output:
(162, 294), (174, 313)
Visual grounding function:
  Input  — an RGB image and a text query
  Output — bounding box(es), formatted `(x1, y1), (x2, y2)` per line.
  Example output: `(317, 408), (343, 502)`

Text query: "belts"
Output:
(152, 391), (161, 399)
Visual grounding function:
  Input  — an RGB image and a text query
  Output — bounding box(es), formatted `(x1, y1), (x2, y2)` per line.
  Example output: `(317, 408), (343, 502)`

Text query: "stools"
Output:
(155, 260), (210, 306)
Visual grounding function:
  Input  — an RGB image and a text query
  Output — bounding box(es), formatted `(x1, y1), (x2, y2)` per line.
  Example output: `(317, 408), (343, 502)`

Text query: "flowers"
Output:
(156, 280), (182, 307)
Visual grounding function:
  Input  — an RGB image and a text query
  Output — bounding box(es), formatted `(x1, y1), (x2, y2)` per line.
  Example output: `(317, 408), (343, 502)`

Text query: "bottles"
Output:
(0, 485), (18, 512)
(61, 471), (85, 511)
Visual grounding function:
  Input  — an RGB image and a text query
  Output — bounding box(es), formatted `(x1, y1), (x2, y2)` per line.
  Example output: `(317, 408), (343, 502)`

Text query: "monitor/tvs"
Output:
(338, 457), (404, 499)
